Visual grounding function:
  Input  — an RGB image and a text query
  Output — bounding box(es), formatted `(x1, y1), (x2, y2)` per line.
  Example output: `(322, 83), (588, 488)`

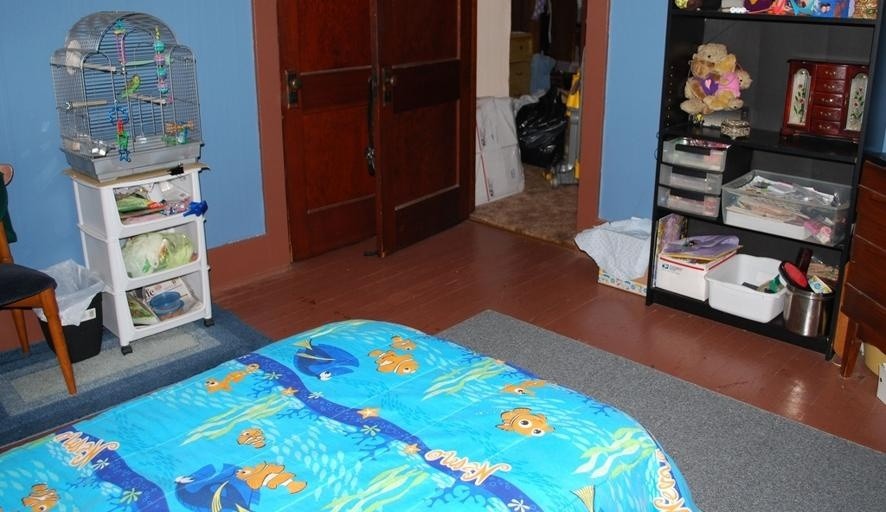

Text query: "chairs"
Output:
(0, 162), (76, 397)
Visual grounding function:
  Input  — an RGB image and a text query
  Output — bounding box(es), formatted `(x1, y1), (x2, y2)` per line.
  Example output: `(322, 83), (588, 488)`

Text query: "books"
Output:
(660, 233), (745, 272)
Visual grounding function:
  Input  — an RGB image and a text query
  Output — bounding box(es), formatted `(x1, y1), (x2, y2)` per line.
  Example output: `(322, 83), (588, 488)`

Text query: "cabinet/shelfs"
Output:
(63, 166), (213, 355)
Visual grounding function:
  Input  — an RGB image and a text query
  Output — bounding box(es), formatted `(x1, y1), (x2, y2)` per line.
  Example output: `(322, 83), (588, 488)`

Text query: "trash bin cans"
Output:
(33, 257), (106, 365)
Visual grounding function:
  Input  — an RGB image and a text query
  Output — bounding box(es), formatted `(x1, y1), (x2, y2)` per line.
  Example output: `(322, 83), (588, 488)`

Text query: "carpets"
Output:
(1, 301), (276, 450)
(428, 307), (885, 512)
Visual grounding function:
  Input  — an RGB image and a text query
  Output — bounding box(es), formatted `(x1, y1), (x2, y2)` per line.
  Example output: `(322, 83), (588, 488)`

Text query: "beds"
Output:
(1, 316), (700, 512)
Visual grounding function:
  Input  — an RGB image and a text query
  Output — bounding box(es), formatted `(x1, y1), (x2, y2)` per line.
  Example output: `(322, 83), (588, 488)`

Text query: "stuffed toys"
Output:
(680, 41), (753, 113)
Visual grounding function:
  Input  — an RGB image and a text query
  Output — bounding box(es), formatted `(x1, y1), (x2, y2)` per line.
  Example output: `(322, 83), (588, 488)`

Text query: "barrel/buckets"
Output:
(783, 284), (833, 340)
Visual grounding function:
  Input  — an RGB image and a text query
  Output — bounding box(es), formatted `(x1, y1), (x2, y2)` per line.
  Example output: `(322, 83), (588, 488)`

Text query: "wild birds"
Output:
(117, 75), (141, 99)
